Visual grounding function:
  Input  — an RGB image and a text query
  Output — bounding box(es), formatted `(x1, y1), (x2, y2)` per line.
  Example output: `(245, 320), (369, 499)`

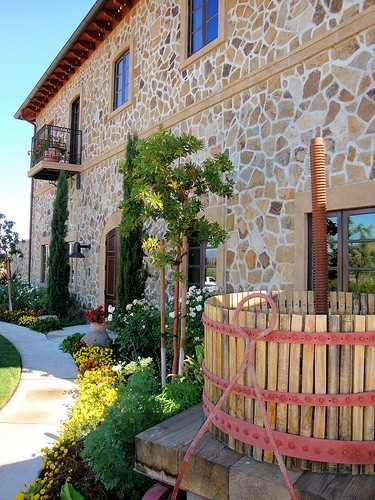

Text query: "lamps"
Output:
(69, 242), (91, 259)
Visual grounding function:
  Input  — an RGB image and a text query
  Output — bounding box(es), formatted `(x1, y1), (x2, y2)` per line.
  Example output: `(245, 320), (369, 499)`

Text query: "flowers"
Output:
(84, 306), (107, 324)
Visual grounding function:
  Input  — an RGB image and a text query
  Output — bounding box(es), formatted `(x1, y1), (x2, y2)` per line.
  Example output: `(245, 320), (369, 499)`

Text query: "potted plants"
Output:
(39, 135), (67, 163)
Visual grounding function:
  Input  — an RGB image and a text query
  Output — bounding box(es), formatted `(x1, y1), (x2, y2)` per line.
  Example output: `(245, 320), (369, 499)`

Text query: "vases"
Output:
(90, 323), (105, 332)
